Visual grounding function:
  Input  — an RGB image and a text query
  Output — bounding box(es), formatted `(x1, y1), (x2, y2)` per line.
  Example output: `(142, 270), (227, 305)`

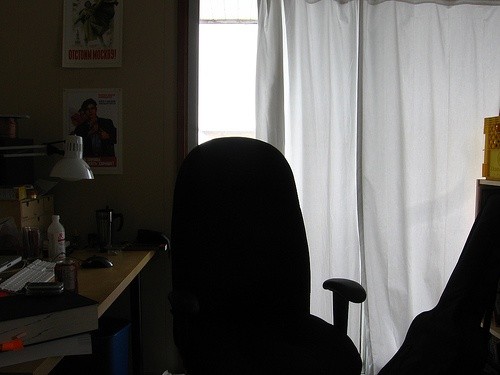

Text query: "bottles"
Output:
(47, 214), (66, 264)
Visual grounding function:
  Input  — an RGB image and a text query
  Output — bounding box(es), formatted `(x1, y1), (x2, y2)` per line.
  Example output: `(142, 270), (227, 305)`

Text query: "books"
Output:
(0, 291), (99, 368)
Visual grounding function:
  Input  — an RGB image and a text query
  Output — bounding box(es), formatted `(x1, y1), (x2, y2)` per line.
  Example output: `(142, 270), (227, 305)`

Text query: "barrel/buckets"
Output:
(91, 319), (131, 375)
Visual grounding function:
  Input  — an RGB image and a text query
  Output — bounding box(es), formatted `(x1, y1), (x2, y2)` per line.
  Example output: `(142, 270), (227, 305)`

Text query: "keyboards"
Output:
(0, 259), (56, 292)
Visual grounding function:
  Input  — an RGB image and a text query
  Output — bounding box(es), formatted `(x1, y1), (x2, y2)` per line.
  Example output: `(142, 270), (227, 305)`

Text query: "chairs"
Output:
(166, 136), (367, 375)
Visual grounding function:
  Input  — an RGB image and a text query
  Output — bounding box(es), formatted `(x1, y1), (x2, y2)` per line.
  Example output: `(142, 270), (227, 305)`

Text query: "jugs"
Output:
(96, 205), (124, 250)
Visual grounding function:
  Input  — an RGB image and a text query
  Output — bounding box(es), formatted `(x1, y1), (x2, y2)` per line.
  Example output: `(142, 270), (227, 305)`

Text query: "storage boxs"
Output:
(483, 116), (499, 182)
(0, 188), (56, 253)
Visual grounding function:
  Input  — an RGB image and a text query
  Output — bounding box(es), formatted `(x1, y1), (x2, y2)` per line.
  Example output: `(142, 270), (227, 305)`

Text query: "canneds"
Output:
(54, 260), (77, 296)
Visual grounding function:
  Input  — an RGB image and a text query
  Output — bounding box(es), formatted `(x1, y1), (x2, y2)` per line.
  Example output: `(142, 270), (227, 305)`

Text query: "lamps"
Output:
(0, 135), (95, 180)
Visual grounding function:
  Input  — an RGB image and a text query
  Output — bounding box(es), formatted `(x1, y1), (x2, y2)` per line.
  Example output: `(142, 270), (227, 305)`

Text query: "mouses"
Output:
(80, 255), (114, 269)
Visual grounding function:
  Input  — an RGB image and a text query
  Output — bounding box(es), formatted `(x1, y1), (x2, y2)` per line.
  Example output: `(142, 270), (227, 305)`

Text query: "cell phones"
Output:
(27, 282), (64, 294)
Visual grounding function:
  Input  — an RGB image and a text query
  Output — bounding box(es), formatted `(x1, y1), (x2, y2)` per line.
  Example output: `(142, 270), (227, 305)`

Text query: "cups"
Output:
(23, 225), (42, 258)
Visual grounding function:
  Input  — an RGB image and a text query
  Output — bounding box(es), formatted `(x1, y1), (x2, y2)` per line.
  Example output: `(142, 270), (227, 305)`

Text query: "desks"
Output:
(0, 249), (155, 374)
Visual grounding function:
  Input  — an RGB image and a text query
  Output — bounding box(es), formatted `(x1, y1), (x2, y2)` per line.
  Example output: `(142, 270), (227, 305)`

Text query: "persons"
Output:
(74, 99), (115, 155)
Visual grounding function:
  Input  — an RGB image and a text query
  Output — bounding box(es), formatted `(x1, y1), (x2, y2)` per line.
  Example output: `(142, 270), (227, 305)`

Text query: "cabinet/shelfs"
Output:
(477, 180), (500, 375)
